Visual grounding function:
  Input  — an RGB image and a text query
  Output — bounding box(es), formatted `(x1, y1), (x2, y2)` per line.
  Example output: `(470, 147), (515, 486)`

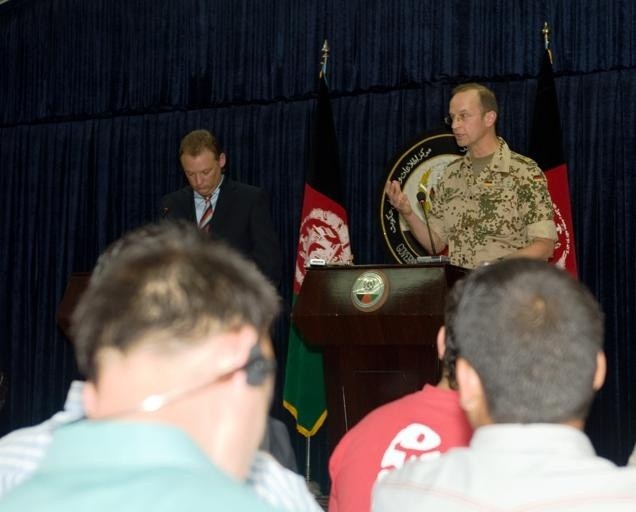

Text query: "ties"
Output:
(197, 194), (212, 235)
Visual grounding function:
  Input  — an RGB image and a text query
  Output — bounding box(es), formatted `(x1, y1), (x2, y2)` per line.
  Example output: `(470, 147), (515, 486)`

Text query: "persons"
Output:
(156, 130), (282, 278)
(385, 83), (558, 270)
(370, 257), (636, 512)
(0, 238), (286, 512)
(0, 219), (326, 512)
(326, 278), (474, 512)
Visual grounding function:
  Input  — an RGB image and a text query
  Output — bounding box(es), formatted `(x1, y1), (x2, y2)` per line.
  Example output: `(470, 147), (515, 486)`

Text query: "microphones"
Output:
(416, 190), (439, 255)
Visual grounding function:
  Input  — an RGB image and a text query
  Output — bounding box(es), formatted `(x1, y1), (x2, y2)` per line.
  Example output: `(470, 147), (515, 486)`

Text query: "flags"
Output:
(283, 42), (356, 437)
(530, 31), (579, 280)
